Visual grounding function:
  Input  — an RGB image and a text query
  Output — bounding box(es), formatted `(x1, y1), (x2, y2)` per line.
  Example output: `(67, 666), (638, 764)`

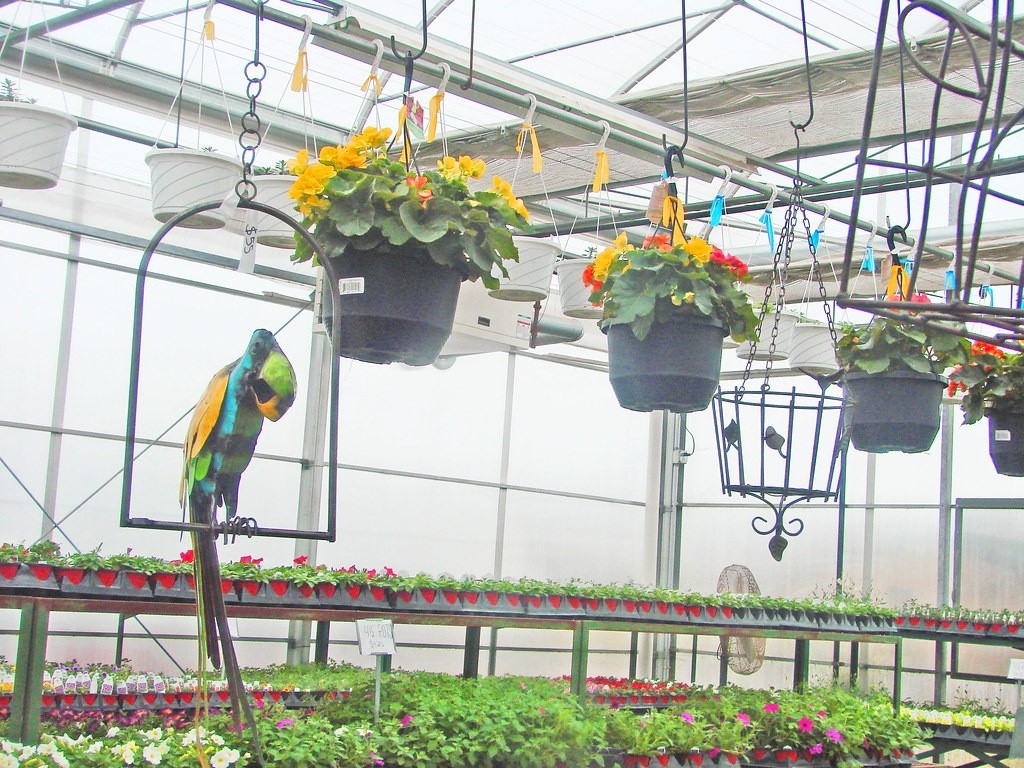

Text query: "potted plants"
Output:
(735, 298), (804, 361)
(788, 309), (858, 378)
(245, 159), (316, 249)
(399, 572), (1024, 648)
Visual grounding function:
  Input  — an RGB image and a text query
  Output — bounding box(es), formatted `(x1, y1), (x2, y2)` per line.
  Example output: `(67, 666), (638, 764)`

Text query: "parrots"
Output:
(179, 329), (298, 768)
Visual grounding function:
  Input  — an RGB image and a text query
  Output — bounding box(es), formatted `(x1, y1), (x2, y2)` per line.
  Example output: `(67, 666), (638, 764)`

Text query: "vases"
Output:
(984, 407), (1024, 476)
(318, 244), (462, 365)
(554, 256), (613, 320)
(597, 313), (731, 413)
(838, 371), (949, 454)
(0, 98), (80, 192)
(145, 147), (254, 230)
(3, 559), (394, 612)
(482, 234), (562, 303)
(0, 689), (358, 713)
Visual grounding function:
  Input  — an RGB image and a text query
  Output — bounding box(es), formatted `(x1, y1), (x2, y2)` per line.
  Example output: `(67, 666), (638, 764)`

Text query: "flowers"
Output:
(833, 288), (1024, 425)
(175, 544), (933, 768)
(287, 113), (536, 285)
(582, 230), (777, 345)
